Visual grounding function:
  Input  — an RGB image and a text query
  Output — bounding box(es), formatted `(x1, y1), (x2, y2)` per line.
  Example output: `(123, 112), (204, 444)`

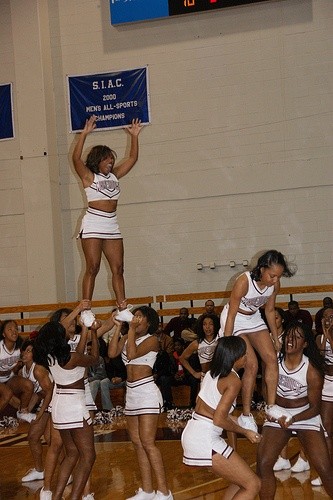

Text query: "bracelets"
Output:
(291, 416), (296, 422)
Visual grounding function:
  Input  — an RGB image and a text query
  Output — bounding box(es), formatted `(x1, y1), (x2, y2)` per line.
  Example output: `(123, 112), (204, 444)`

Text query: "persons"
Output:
(107, 306), (173, 500)
(217, 250), (294, 441)
(0, 297), (333, 500)
(71, 112), (151, 326)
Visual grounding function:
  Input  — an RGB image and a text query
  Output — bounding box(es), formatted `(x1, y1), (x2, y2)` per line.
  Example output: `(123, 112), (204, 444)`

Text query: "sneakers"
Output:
(273, 454), (291, 471)
(126, 487), (156, 500)
(238, 412), (259, 438)
(114, 303), (135, 322)
(151, 489), (174, 500)
(66, 474), (73, 486)
(290, 455), (311, 473)
(78, 308), (97, 327)
(21, 468), (45, 482)
(265, 404), (292, 422)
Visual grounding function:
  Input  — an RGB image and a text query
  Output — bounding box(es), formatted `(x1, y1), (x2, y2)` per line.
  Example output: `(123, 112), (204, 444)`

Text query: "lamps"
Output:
(229, 260), (236, 268)
(196, 263), (203, 270)
(209, 261), (216, 269)
(242, 259), (248, 266)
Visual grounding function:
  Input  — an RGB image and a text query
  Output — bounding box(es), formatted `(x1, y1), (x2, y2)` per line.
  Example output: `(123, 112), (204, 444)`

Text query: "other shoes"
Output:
(82, 491), (95, 500)
(310, 475), (323, 486)
(39, 486), (52, 500)
(16, 409), (36, 423)
